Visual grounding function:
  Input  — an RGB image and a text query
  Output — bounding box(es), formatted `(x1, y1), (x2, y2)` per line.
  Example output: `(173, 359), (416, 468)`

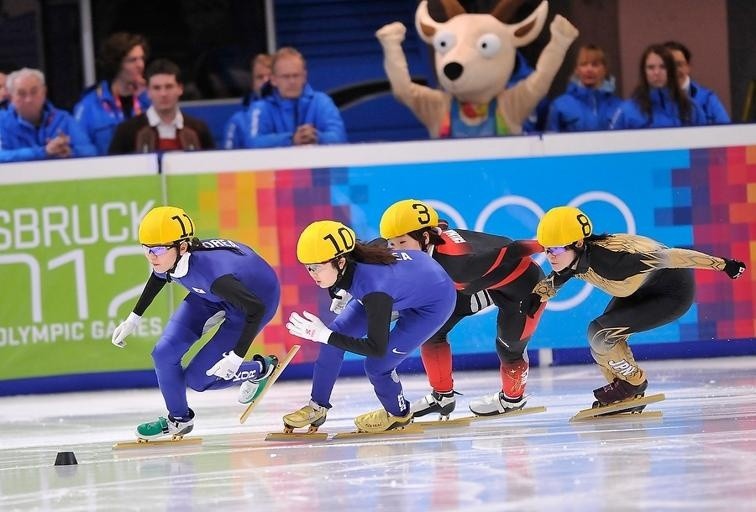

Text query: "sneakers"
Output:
(594, 377), (647, 404)
(238, 353), (279, 404)
(409, 391), (462, 417)
(469, 390), (528, 415)
(135, 408), (195, 440)
(283, 400), (327, 428)
(592, 394), (645, 416)
(354, 409), (412, 432)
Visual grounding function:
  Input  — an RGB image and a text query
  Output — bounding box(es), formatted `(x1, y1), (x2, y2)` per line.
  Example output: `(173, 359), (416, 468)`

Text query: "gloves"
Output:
(206, 350), (245, 380)
(520, 293), (542, 319)
(285, 310), (333, 344)
(329, 290), (353, 315)
(723, 252), (746, 280)
(111, 312), (142, 348)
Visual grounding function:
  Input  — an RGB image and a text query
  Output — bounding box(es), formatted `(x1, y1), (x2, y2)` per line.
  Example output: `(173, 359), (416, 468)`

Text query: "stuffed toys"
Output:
(375, 0), (580, 138)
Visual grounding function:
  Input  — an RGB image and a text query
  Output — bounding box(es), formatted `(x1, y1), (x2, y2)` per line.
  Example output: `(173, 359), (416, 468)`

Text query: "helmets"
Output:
(139, 206), (195, 245)
(537, 207), (594, 247)
(296, 219), (356, 265)
(379, 199), (438, 240)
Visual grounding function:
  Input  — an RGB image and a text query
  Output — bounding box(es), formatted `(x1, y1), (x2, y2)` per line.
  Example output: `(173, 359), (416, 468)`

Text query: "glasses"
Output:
(305, 255), (339, 271)
(141, 239), (186, 256)
(544, 248), (571, 255)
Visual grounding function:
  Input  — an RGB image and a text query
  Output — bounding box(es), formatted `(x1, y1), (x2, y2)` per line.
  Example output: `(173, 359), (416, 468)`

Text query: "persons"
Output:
(520, 206), (746, 405)
(665, 41), (730, 124)
(0, 73), (13, 109)
(222, 54), (275, 150)
(282, 219), (457, 434)
(610, 45), (706, 128)
(111, 206), (280, 441)
(547, 43), (623, 132)
(378, 198), (547, 416)
(0, 69), (98, 162)
(74, 30), (151, 153)
(246, 47), (347, 148)
(108, 60), (216, 155)
